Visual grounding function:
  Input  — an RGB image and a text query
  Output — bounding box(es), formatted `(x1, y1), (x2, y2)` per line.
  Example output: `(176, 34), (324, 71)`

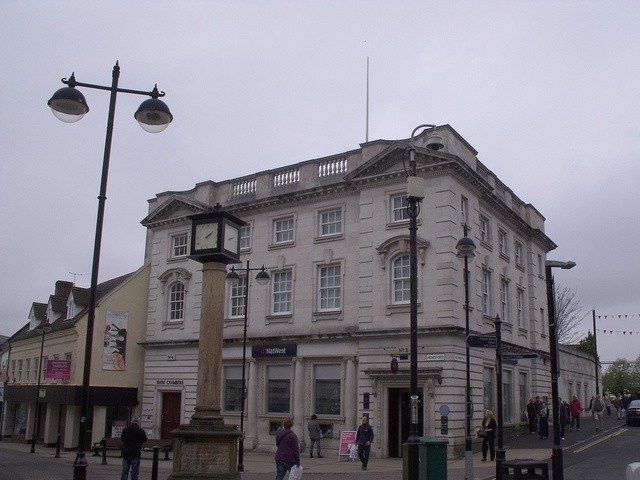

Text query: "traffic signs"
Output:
(502, 353), (540, 359)
(465, 334), (497, 349)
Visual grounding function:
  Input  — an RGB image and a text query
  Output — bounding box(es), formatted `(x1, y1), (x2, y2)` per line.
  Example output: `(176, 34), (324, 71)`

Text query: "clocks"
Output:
(194, 222), (218, 250)
(224, 223), (238, 255)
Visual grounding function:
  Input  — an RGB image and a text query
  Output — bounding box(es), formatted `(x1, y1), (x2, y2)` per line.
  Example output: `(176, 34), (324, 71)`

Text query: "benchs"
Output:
(91, 437), (175, 460)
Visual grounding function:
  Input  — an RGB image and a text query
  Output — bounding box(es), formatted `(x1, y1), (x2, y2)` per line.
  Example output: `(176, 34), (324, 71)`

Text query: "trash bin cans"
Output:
(419, 437), (449, 480)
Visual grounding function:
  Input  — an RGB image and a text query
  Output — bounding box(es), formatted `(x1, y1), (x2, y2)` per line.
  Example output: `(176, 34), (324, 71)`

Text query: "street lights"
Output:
(457, 224), (477, 453)
(46, 61), (172, 480)
(404, 146), (426, 436)
(226, 260), (270, 472)
(545, 260), (576, 480)
(30, 318), (52, 454)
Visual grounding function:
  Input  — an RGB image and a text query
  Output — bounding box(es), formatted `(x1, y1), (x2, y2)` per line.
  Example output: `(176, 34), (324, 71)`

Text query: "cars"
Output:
(626, 400), (640, 426)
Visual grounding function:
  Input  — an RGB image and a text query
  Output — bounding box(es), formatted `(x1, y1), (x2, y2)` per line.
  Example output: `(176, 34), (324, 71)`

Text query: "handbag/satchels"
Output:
(477, 426), (488, 437)
(289, 465), (304, 480)
(349, 445), (360, 459)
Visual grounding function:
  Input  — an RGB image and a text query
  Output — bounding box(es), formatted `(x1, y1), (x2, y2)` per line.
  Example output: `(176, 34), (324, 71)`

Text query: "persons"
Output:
(562, 399), (571, 432)
(539, 402), (549, 440)
(120, 417), (147, 480)
(307, 414), (323, 458)
(589, 396), (595, 421)
(527, 398), (537, 435)
(481, 410), (497, 461)
(274, 417), (300, 479)
(569, 396), (581, 431)
(354, 414), (374, 470)
(534, 395), (542, 431)
(604, 393), (638, 420)
(558, 397), (568, 441)
(591, 395), (606, 432)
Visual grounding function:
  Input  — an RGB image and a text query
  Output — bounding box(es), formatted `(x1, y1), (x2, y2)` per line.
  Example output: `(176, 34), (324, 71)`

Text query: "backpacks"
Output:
(613, 399), (620, 408)
(593, 399), (603, 412)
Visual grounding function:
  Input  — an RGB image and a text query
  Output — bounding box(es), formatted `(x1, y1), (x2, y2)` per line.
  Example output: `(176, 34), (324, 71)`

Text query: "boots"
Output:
(317, 452), (323, 458)
(310, 453), (314, 457)
